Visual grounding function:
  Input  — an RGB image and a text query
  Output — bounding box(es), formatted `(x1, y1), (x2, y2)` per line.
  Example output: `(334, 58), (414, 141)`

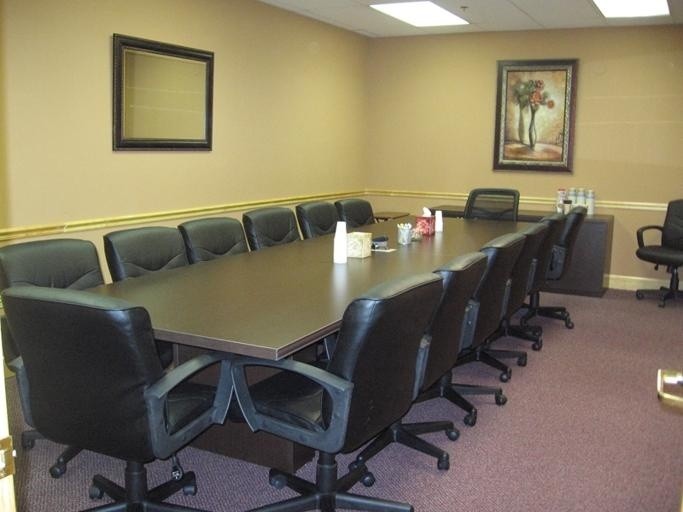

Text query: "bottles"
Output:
(567, 188), (577, 207)
(562, 199), (571, 214)
(557, 187), (565, 213)
(576, 188), (585, 206)
(586, 190), (595, 215)
(435, 211), (443, 234)
(333, 220), (348, 263)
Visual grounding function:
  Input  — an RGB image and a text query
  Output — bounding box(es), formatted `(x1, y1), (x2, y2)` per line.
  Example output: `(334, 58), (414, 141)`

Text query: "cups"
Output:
(397, 226), (413, 245)
(553, 204), (563, 214)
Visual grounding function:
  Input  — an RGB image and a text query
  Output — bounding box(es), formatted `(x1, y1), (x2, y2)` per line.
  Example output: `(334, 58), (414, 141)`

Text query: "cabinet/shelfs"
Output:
(427, 203), (615, 298)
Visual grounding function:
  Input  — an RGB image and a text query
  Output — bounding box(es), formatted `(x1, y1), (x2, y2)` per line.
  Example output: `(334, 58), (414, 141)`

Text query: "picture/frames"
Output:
(491, 55), (581, 174)
(110, 32), (215, 152)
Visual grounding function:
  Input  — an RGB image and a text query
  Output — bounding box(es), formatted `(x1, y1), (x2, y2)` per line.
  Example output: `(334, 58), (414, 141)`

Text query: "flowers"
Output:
(518, 79), (556, 114)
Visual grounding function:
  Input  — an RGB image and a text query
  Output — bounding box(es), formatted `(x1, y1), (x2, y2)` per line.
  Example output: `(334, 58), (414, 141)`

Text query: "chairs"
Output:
(633, 199), (683, 309)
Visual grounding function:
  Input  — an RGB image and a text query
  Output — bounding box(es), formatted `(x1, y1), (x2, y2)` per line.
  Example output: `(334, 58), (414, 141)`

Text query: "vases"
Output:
(529, 111), (537, 150)
